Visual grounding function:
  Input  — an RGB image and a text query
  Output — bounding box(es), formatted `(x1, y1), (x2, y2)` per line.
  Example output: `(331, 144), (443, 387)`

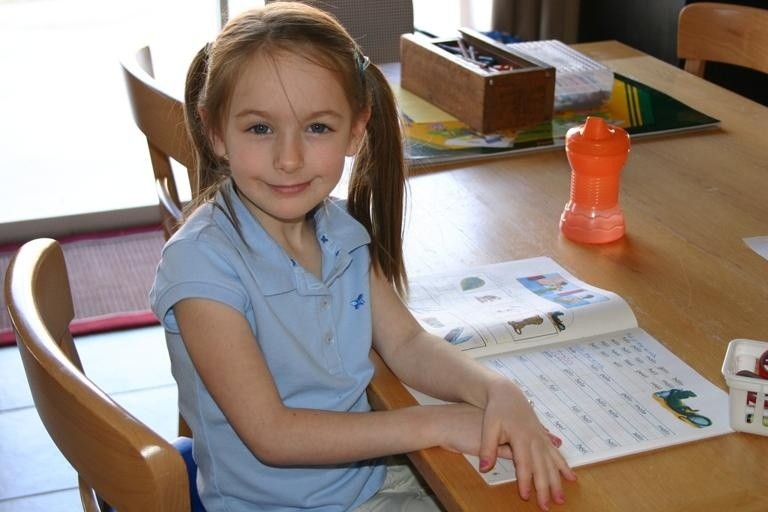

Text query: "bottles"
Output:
(559, 115), (632, 245)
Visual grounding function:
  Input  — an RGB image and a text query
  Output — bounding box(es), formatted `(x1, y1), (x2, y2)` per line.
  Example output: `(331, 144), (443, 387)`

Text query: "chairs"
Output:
(4, 237), (205, 512)
(676, 2), (768, 78)
(119, 45), (197, 240)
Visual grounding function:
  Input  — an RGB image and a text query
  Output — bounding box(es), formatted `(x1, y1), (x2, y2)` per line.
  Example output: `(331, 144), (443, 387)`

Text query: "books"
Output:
(392, 254), (739, 486)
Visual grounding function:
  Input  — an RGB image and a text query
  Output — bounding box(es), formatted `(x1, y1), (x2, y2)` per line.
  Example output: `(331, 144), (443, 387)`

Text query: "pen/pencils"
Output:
(438, 36), (513, 73)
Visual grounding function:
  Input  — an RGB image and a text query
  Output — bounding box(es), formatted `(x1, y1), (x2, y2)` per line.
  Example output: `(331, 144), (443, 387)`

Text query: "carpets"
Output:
(0, 223), (166, 346)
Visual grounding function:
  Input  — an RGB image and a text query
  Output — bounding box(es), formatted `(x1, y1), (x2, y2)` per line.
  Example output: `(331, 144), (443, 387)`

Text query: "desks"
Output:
(226, 39), (768, 512)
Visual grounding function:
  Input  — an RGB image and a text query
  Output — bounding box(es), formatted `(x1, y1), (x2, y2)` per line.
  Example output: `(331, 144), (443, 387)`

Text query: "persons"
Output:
(147, 1), (578, 512)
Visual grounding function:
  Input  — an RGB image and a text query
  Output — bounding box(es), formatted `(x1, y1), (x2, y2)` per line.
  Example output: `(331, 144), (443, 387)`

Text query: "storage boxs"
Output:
(401, 28), (556, 135)
